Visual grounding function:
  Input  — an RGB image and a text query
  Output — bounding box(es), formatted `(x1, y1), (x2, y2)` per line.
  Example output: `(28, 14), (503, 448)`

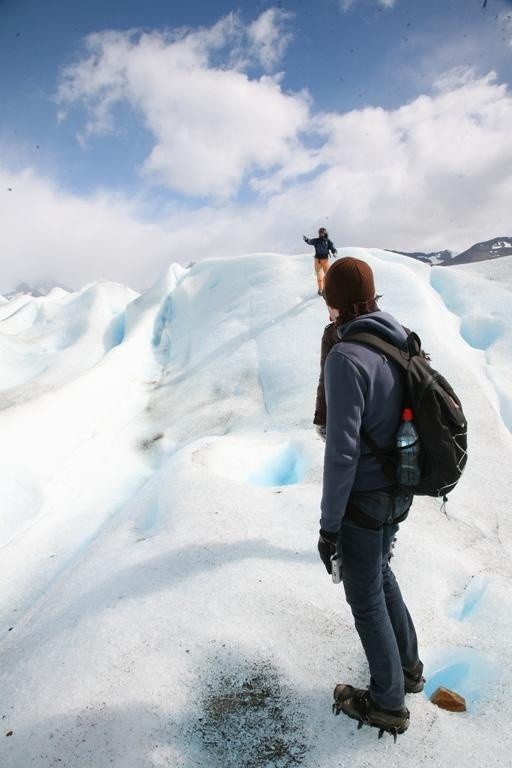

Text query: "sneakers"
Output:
(403, 659), (426, 693)
(333, 684), (411, 744)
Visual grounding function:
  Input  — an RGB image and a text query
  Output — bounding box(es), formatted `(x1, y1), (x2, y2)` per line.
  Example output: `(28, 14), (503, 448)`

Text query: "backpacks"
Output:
(334, 326), (468, 499)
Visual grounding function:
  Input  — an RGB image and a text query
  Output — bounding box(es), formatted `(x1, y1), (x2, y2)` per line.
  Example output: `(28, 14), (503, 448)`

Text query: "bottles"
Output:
(396, 406), (423, 488)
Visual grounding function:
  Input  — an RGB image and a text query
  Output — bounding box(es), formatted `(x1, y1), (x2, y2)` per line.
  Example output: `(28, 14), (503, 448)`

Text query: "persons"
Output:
(313, 256), (430, 737)
(301, 227), (338, 296)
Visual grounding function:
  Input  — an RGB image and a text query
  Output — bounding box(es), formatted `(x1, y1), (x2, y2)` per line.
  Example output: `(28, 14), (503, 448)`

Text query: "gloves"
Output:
(317, 529), (337, 574)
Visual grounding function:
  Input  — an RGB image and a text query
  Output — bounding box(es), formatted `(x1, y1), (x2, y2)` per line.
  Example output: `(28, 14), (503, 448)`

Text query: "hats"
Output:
(322, 257), (375, 309)
